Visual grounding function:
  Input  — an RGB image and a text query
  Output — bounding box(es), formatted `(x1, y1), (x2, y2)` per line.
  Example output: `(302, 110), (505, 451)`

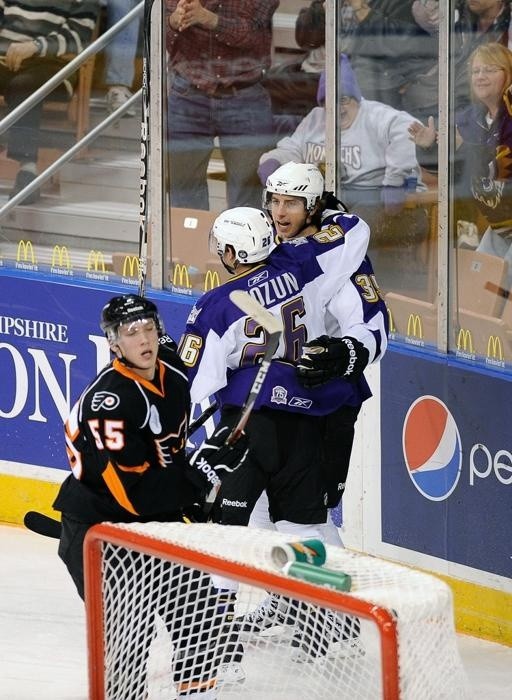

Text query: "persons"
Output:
(256, 53), (431, 252)
(175, 206), (371, 687)
(52, 292), (257, 699)
(0, 0), (102, 206)
(435, 83), (512, 183)
(165, 0), (280, 218)
(235, 160), (392, 645)
(294, 0), (437, 109)
(102, 0), (141, 119)
(411, 0), (512, 190)
(407, 43), (512, 319)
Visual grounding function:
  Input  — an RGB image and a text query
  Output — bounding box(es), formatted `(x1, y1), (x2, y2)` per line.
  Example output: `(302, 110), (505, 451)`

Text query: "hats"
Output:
(316, 56), (362, 107)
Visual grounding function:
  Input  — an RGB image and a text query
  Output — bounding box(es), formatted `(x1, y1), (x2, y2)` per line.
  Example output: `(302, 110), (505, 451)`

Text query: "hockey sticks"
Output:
(23, 400), (221, 540)
(204, 289), (284, 512)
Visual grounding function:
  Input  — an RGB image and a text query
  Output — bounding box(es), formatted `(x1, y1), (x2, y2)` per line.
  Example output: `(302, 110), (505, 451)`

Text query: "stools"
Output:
(0, 18), (98, 167)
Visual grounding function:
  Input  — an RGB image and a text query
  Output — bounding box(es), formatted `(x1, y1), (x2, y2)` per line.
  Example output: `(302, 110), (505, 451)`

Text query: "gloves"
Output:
(256, 159), (280, 187)
(294, 334), (370, 390)
(379, 185), (407, 218)
(179, 424), (255, 497)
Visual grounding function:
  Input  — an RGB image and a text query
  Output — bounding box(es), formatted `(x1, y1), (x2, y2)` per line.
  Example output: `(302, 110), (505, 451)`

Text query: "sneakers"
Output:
(235, 602), (293, 632)
(104, 87), (137, 120)
(291, 613), (361, 648)
(7, 169), (42, 206)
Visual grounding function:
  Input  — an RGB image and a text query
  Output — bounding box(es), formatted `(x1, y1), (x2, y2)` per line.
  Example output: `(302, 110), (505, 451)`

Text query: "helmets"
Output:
(98, 293), (166, 345)
(207, 207), (276, 267)
(263, 161), (325, 211)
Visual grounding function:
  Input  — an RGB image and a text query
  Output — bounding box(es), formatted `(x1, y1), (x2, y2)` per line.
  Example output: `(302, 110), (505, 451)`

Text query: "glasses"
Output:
(470, 66), (504, 74)
(340, 95), (354, 104)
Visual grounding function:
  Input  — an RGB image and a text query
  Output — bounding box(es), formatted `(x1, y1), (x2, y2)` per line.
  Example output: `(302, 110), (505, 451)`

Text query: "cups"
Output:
(268, 539), (326, 572)
